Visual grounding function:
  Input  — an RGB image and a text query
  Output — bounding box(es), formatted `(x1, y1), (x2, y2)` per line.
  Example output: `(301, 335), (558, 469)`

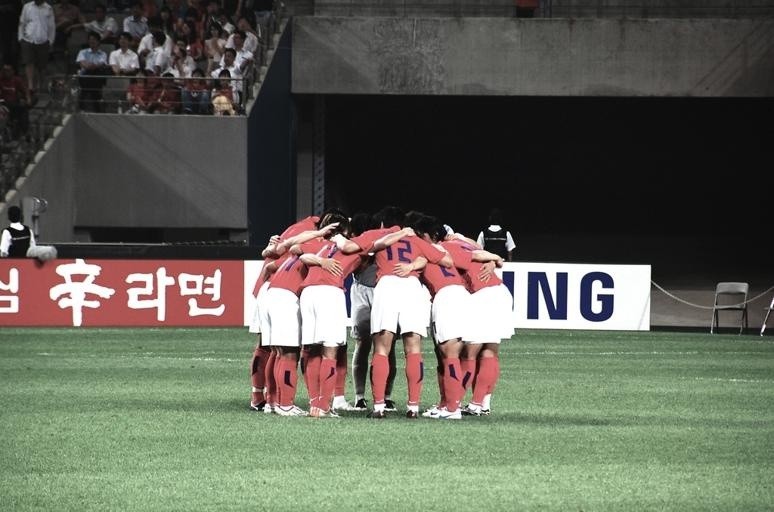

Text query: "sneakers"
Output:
(367, 400), (397, 418)
(250, 398), (343, 418)
(405, 404), (490, 420)
(332, 398), (368, 411)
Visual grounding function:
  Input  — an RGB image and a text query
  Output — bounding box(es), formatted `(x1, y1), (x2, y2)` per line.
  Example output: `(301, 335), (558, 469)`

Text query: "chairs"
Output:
(759, 297), (774, 337)
(64, 1), (282, 111)
(709, 281), (750, 337)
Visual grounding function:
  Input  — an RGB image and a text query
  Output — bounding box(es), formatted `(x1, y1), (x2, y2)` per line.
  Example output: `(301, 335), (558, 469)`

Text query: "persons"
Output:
(0, 206), (36, 258)
(0, 1), (273, 134)
(515, 0), (539, 18)
(250, 204), (515, 419)
